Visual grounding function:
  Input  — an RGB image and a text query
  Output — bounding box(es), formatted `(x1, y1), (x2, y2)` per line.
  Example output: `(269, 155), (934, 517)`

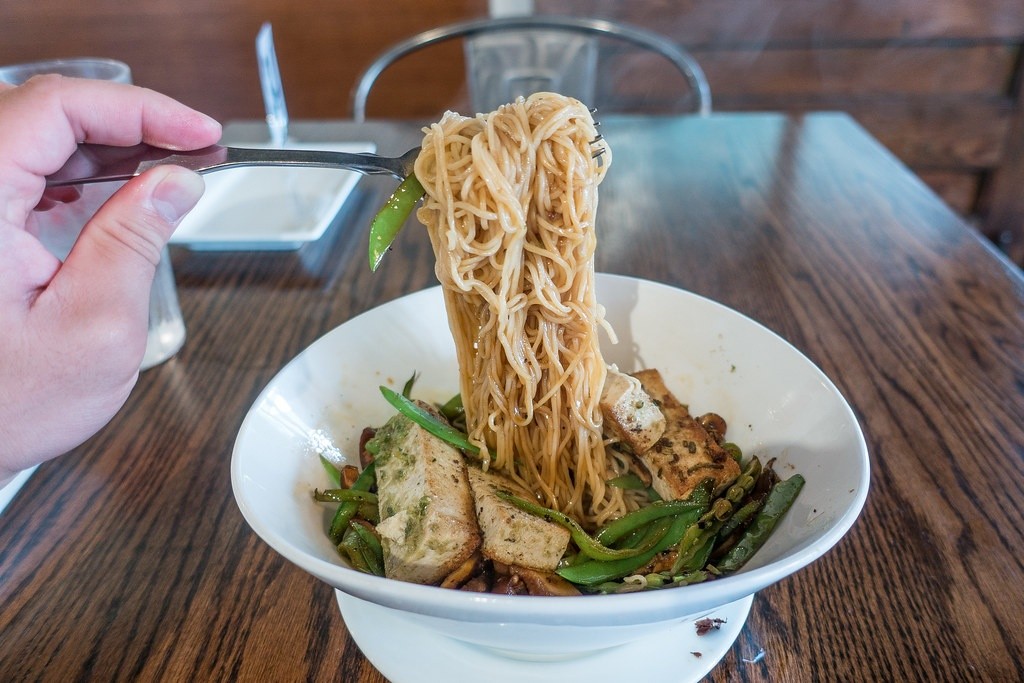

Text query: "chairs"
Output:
(349, 16), (711, 126)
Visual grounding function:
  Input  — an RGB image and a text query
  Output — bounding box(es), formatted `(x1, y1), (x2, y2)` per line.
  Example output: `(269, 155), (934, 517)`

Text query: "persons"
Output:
(0, 73), (223, 492)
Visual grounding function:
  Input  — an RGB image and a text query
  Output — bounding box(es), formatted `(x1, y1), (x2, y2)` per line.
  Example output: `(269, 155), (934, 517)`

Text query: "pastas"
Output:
(413, 90), (649, 534)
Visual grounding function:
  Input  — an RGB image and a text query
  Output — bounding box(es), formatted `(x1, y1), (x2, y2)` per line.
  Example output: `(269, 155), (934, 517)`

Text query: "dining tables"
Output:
(0, 106), (1024, 683)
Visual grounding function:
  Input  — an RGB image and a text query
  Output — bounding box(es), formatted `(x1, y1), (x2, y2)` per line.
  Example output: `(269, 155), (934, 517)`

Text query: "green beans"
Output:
(312, 386), (806, 596)
(368, 172), (424, 274)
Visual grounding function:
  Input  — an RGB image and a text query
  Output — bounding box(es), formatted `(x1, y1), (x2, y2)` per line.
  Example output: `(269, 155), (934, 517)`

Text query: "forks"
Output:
(46, 106), (606, 200)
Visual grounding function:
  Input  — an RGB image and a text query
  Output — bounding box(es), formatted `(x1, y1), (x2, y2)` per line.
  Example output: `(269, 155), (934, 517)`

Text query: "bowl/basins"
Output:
(230, 273), (872, 664)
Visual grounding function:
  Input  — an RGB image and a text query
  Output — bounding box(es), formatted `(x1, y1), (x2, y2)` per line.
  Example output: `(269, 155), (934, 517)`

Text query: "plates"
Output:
(335, 589), (754, 683)
(166, 141), (376, 251)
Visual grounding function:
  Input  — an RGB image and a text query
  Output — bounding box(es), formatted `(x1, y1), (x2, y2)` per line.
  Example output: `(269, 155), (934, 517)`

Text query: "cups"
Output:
(0, 57), (186, 372)
